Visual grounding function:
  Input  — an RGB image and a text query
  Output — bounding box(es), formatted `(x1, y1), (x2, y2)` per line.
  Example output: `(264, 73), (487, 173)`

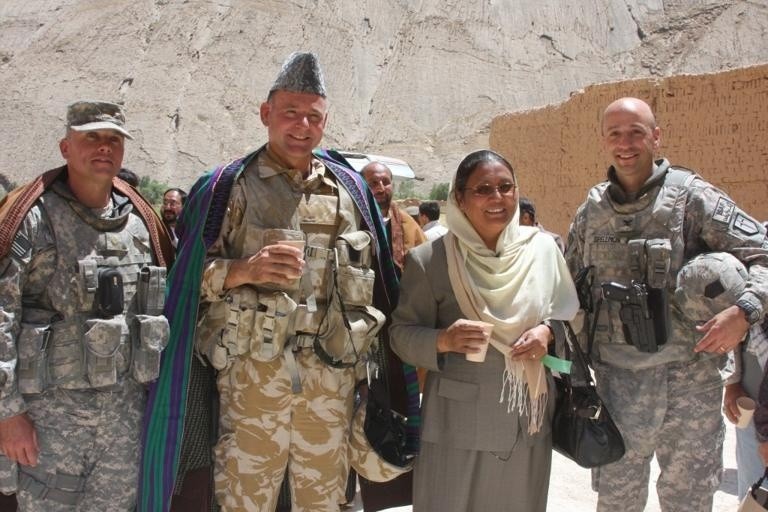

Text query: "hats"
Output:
(66, 101), (132, 141)
(270, 51), (327, 97)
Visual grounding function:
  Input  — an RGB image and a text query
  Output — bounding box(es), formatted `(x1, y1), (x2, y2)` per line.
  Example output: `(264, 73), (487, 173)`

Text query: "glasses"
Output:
(462, 183), (516, 197)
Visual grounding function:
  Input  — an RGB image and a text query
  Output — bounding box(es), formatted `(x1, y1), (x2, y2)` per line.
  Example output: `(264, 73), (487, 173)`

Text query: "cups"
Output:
(277, 239), (306, 280)
(466, 321), (494, 363)
(734, 397), (755, 430)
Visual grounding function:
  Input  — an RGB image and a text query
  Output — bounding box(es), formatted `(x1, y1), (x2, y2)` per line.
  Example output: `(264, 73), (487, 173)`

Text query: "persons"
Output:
(519, 195), (564, 257)
(564, 98), (767, 510)
(385, 149), (580, 511)
(359, 161), (428, 269)
(0, 100), (173, 511)
(417, 199), (449, 242)
(159, 186), (189, 250)
(722, 316), (767, 505)
(173, 48), (388, 512)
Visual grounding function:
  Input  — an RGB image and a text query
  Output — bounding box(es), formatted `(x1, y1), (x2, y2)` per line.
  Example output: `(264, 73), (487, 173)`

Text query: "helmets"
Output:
(674, 248), (749, 323)
(348, 396), (416, 484)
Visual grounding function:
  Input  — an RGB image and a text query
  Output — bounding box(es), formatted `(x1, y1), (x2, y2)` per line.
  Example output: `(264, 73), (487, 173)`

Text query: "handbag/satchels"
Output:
(552, 383), (625, 468)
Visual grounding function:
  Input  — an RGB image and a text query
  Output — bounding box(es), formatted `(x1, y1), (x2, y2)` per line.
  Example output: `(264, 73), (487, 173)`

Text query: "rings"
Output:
(533, 353), (536, 359)
(720, 346), (725, 352)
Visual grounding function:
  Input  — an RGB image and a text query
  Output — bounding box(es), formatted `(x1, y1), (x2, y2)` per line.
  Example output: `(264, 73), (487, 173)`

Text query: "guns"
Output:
(601, 280), (658, 354)
